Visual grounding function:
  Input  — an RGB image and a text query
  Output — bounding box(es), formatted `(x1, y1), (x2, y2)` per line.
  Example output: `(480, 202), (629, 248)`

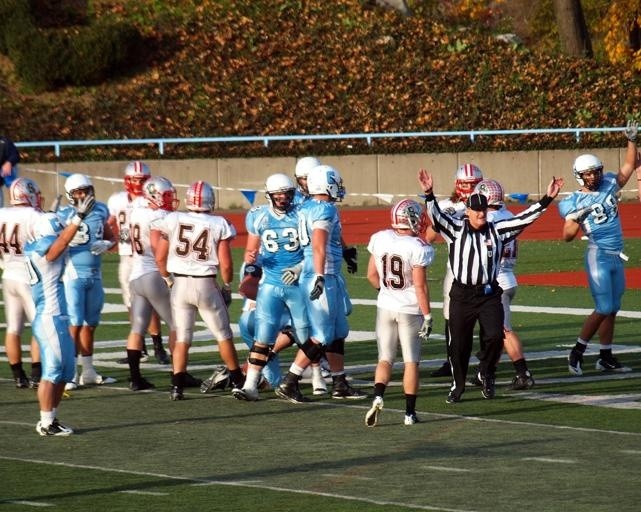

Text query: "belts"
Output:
(173, 273), (216, 277)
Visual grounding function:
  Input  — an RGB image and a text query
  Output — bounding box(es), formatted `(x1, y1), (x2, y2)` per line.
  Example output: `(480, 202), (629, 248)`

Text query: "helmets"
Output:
(265, 157), (345, 213)
(572, 155), (602, 191)
(455, 164), (503, 210)
(391, 199), (425, 235)
(124, 161), (180, 211)
(185, 180), (214, 211)
(65, 174), (95, 205)
(10, 178), (44, 211)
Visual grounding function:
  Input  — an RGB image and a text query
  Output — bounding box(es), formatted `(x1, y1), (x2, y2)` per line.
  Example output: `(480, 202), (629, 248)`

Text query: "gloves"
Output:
(281, 267), (300, 285)
(162, 274), (173, 288)
(419, 318), (432, 339)
(222, 287), (231, 306)
(310, 276), (324, 300)
(77, 195), (94, 219)
(572, 209), (594, 224)
(343, 248), (357, 273)
(91, 240), (116, 255)
(625, 120), (638, 142)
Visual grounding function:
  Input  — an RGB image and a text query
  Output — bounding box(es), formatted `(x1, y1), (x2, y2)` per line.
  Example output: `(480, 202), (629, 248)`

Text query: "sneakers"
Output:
(568, 352), (582, 376)
(405, 414), (419, 425)
(430, 362), (534, 403)
(597, 358), (632, 372)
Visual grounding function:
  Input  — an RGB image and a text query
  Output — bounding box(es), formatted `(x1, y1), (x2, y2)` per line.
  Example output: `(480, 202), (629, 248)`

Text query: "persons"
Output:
(1, 135), (20, 204)
(557, 118), (641, 378)
(235, 151), (366, 400)
(425, 159), (535, 395)
(366, 198), (437, 428)
(101, 160), (173, 366)
(2, 175), (51, 389)
(126, 171), (202, 395)
(42, 175), (114, 397)
(23, 191), (97, 438)
(418, 167), (566, 404)
(156, 175), (246, 402)
